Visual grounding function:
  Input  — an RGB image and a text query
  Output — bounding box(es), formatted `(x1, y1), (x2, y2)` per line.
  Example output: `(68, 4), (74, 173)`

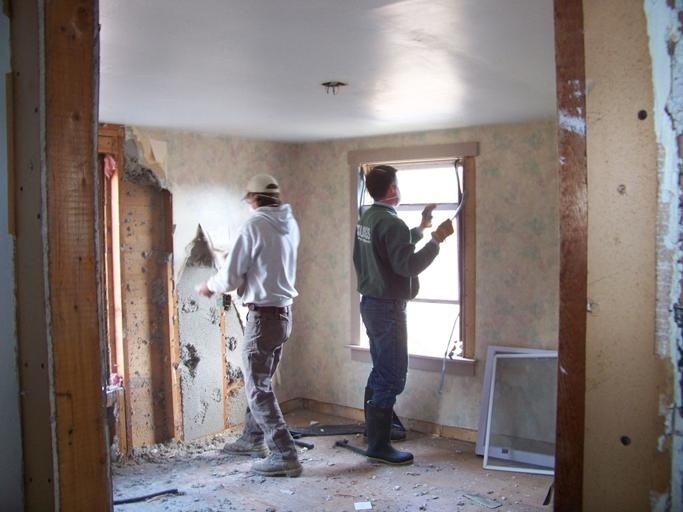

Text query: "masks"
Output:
(395, 186), (402, 208)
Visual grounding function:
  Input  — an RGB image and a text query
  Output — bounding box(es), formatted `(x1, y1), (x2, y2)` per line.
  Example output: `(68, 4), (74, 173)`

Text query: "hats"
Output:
(238, 172), (281, 202)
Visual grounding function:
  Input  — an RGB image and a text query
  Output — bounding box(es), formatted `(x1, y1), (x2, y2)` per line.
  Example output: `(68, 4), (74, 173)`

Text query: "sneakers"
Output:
(221, 438), (271, 459)
(249, 454), (305, 479)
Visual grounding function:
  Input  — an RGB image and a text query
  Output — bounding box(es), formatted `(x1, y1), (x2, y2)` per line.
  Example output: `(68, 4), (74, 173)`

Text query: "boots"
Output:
(362, 386), (415, 466)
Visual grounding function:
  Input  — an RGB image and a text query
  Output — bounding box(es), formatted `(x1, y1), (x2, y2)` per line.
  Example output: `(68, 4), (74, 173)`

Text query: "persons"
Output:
(350, 161), (454, 464)
(193, 174), (303, 477)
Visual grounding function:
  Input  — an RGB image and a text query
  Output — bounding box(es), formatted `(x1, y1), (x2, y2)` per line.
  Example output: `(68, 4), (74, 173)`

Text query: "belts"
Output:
(247, 302), (292, 314)
